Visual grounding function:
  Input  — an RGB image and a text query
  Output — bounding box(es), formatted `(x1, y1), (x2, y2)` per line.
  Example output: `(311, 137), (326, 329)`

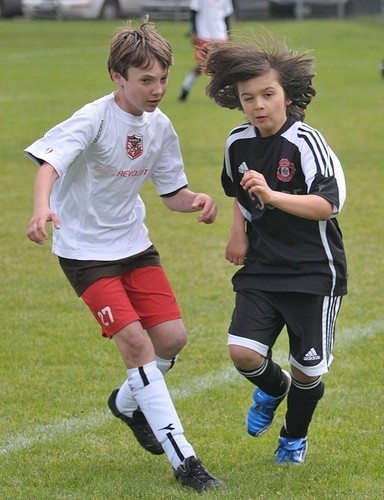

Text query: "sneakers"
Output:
(274, 427), (308, 462)
(108, 388), (165, 455)
(248, 369), (292, 437)
(173, 456), (227, 492)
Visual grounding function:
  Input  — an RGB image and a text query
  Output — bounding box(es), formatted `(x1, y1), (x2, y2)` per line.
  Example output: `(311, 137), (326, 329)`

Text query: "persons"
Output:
(204, 43), (348, 465)
(178, 0), (234, 102)
(22, 22), (228, 496)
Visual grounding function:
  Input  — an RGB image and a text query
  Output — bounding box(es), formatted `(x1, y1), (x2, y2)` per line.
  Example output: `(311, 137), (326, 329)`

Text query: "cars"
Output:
(21, 0), (196, 21)
